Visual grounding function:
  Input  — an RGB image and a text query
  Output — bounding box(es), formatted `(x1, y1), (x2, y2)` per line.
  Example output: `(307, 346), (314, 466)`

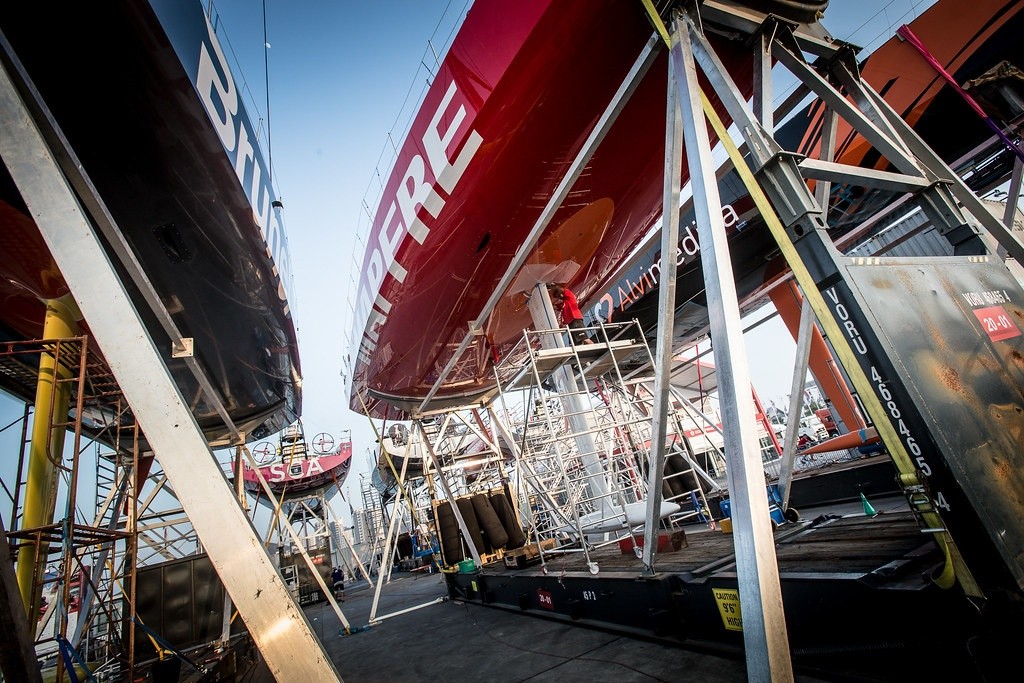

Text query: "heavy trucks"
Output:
(769, 409), (840, 452)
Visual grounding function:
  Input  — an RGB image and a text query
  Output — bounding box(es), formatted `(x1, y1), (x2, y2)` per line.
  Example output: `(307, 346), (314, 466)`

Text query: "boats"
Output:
(370, 431), (482, 501)
(0, 0), (302, 454)
(338, 0), (1022, 422)
(229, 419), (352, 514)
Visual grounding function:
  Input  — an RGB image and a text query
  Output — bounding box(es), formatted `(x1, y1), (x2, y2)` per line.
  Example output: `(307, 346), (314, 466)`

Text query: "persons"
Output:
(414, 523), (429, 549)
(549, 283), (593, 347)
(333, 568), (345, 601)
